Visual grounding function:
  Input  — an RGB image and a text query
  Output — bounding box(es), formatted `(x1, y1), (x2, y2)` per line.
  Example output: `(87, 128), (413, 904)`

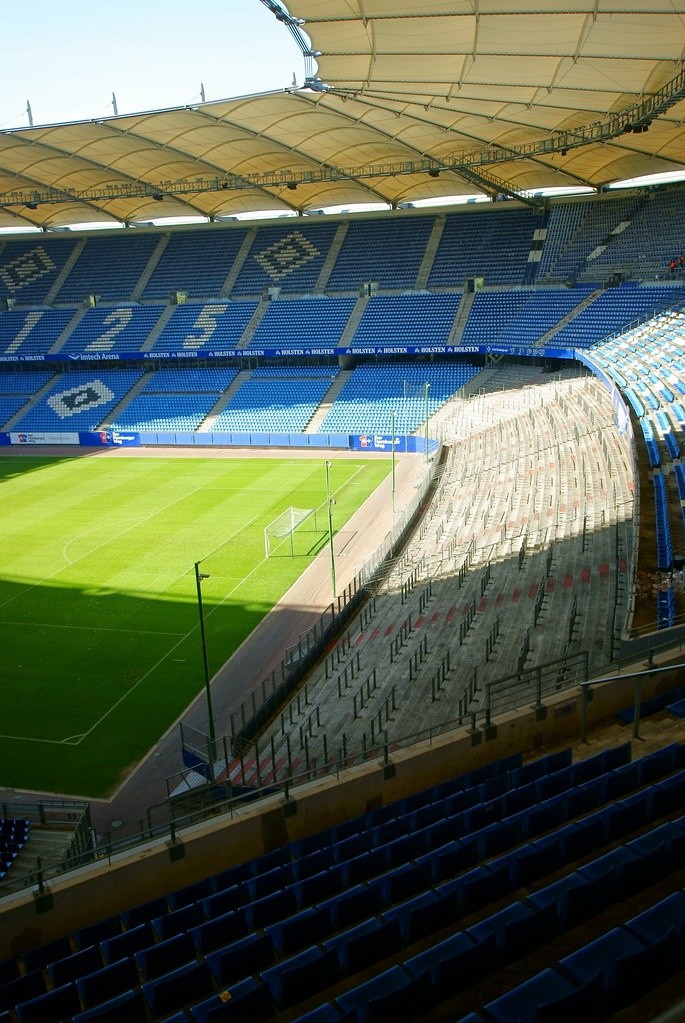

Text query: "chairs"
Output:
(0, 189), (684, 1023)
